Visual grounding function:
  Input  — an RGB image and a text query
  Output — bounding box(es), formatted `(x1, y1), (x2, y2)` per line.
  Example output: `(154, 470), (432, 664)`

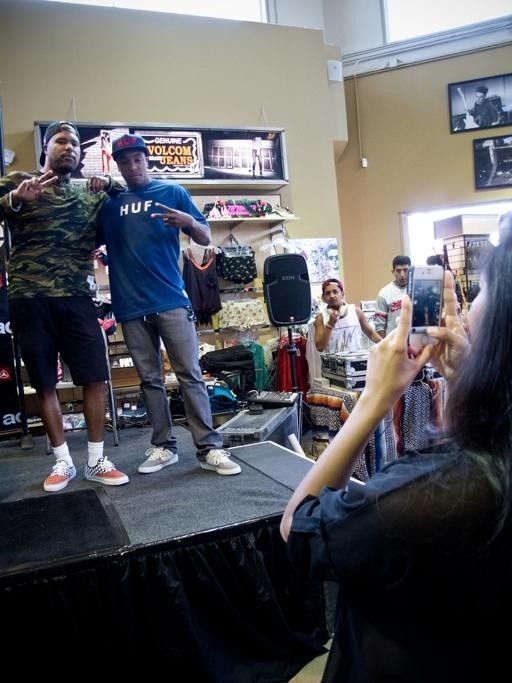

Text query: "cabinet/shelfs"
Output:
(17, 194), (300, 450)
(32, 120), (287, 192)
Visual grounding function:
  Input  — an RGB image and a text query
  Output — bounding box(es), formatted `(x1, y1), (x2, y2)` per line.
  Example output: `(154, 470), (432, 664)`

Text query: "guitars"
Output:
(454, 84), (478, 131)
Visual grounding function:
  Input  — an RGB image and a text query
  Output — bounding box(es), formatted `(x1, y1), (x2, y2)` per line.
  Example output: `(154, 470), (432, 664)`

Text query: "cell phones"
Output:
(406, 265), (445, 347)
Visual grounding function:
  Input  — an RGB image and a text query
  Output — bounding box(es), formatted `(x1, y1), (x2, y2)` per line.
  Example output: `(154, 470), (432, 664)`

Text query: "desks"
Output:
(311, 376), (448, 483)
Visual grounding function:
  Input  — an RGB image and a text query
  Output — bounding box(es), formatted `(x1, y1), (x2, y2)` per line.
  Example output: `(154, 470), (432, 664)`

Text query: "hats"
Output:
(112, 135), (149, 160)
(322, 280), (343, 302)
(40, 121), (79, 164)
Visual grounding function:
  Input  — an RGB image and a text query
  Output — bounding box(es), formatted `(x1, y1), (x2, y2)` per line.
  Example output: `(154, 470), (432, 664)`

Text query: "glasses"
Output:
(326, 256), (338, 260)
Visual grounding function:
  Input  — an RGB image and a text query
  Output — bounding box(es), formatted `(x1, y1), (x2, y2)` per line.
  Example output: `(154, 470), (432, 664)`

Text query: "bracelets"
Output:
(326, 323), (333, 329)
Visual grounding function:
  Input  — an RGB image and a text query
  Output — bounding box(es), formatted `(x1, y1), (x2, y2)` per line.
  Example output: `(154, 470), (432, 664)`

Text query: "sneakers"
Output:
(44, 460), (76, 492)
(85, 457), (129, 485)
(140, 447), (178, 473)
(200, 449), (241, 475)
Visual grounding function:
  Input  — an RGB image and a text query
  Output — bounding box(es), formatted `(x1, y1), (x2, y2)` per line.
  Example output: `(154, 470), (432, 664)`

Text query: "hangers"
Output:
(214, 235), (254, 257)
(258, 227), (309, 261)
(180, 234), (216, 254)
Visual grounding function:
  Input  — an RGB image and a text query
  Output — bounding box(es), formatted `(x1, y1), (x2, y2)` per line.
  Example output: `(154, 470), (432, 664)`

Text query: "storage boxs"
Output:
(214, 401), (300, 452)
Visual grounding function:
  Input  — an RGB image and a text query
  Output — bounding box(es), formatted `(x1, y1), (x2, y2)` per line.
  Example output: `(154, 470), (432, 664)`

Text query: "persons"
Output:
(373, 256), (412, 338)
(1, 120), (130, 492)
(464, 86), (504, 128)
(278, 213), (512, 683)
(93, 134), (241, 476)
(250, 135), (264, 180)
(312, 278), (384, 352)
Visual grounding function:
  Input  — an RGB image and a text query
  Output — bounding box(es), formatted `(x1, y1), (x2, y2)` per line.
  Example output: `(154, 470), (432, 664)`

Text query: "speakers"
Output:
(263, 254), (312, 327)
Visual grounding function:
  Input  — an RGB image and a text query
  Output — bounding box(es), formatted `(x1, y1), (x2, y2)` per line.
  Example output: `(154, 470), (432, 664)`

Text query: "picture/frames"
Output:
(445, 69), (512, 136)
(471, 133), (512, 189)
